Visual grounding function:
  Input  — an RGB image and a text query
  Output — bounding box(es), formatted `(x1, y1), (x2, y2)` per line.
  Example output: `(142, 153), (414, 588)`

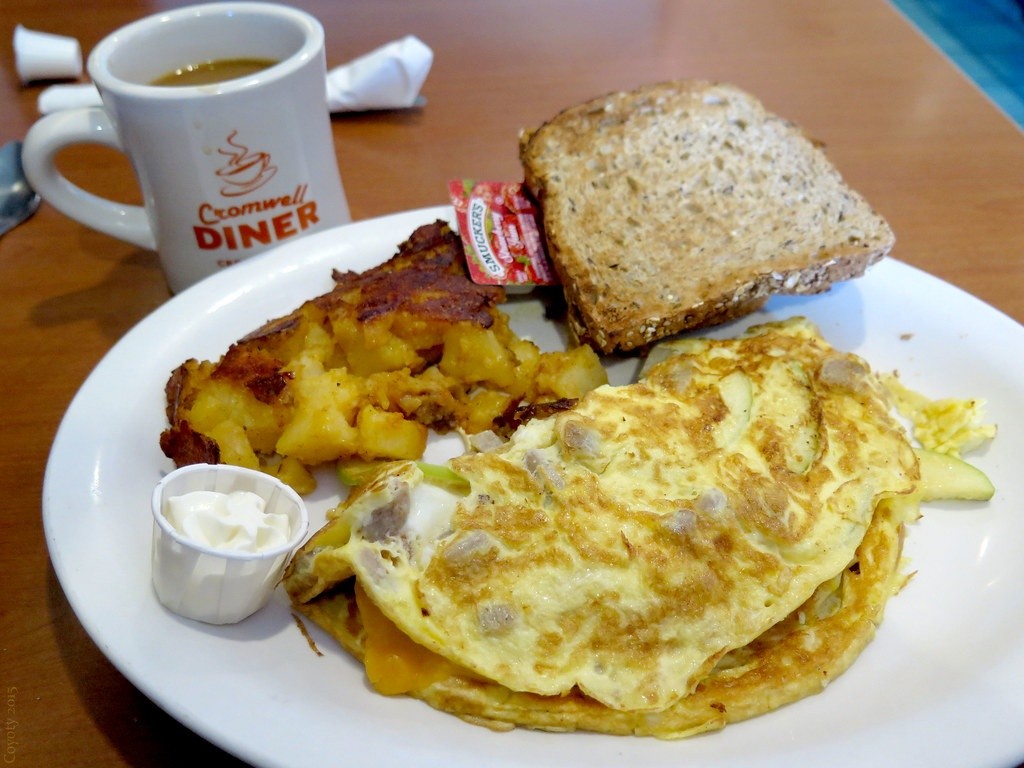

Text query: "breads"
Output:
(520, 75), (897, 354)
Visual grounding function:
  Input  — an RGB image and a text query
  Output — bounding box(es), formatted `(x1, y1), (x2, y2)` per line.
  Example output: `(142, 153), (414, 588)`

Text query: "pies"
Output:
(283, 316), (925, 740)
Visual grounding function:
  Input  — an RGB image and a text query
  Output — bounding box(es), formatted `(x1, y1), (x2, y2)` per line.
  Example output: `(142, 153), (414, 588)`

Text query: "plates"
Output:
(44, 204), (1024, 767)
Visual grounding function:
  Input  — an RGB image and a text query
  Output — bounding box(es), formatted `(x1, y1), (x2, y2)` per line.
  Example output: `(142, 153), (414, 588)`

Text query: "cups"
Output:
(20, 2), (352, 295)
(152, 463), (307, 625)
